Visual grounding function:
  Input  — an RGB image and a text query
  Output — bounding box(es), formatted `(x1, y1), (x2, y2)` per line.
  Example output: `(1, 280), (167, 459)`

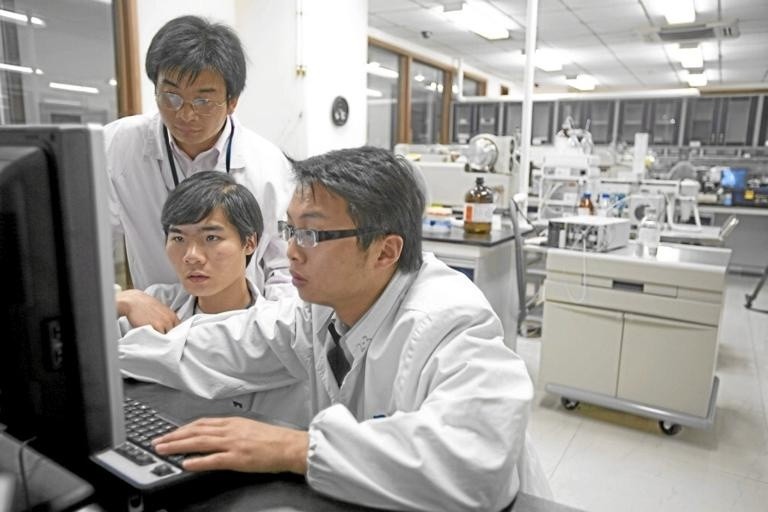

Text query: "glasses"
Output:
(277, 220), (365, 249)
(156, 91), (231, 115)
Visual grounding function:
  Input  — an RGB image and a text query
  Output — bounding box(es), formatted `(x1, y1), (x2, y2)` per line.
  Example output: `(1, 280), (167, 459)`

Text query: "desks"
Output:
(94, 380), (588, 510)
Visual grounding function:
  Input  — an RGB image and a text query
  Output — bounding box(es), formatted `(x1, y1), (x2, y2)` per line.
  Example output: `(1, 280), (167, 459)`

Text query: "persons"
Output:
(117, 146), (554, 512)
(117, 171), (309, 427)
(101, 15), (297, 334)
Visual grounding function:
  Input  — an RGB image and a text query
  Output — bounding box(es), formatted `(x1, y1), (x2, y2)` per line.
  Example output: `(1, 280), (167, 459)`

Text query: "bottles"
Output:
(463, 176), (493, 233)
(579, 192), (623, 218)
(635, 208), (660, 258)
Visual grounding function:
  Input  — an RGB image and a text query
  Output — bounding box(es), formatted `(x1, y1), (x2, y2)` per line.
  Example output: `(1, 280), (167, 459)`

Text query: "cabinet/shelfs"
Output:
(541, 248), (722, 418)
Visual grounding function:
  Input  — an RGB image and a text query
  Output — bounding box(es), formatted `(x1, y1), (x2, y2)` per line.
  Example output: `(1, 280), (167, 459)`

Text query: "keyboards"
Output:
(89, 394), (205, 489)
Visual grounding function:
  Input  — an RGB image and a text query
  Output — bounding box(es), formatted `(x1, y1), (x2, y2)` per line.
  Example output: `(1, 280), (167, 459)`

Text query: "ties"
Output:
(326, 322), (351, 389)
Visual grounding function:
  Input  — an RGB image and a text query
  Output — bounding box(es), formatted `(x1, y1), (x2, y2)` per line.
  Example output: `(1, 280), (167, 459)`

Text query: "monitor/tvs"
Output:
(0, 124), (126, 512)
(719, 167), (747, 192)
